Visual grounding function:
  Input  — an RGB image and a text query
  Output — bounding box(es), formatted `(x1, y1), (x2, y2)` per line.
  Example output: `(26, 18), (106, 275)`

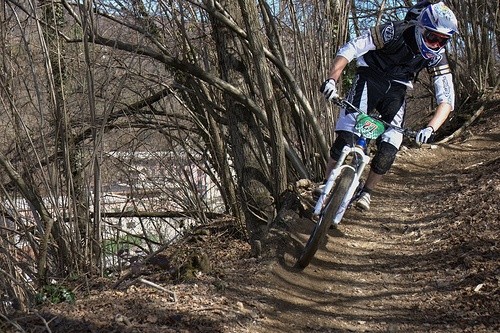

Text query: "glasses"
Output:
(422, 28), (449, 48)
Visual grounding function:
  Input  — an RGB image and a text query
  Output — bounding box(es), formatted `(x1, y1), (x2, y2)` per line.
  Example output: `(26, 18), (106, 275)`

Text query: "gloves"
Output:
(322, 78), (337, 103)
(415, 126), (434, 148)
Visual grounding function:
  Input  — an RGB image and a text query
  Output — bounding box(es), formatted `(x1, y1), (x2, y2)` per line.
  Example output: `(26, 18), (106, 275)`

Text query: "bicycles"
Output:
(296, 83), (438, 267)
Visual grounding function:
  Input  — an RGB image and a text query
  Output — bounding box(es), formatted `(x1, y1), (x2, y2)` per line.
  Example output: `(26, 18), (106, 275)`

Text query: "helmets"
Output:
(415, 2), (458, 38)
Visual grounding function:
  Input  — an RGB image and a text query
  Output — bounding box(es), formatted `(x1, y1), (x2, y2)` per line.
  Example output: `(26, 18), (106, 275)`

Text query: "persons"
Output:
(312, 2), (460, 214)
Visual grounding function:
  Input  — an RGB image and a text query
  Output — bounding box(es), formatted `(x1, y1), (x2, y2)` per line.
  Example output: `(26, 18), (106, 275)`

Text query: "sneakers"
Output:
(311, 187), (324, 203)
(356, 190), (371, 212)
